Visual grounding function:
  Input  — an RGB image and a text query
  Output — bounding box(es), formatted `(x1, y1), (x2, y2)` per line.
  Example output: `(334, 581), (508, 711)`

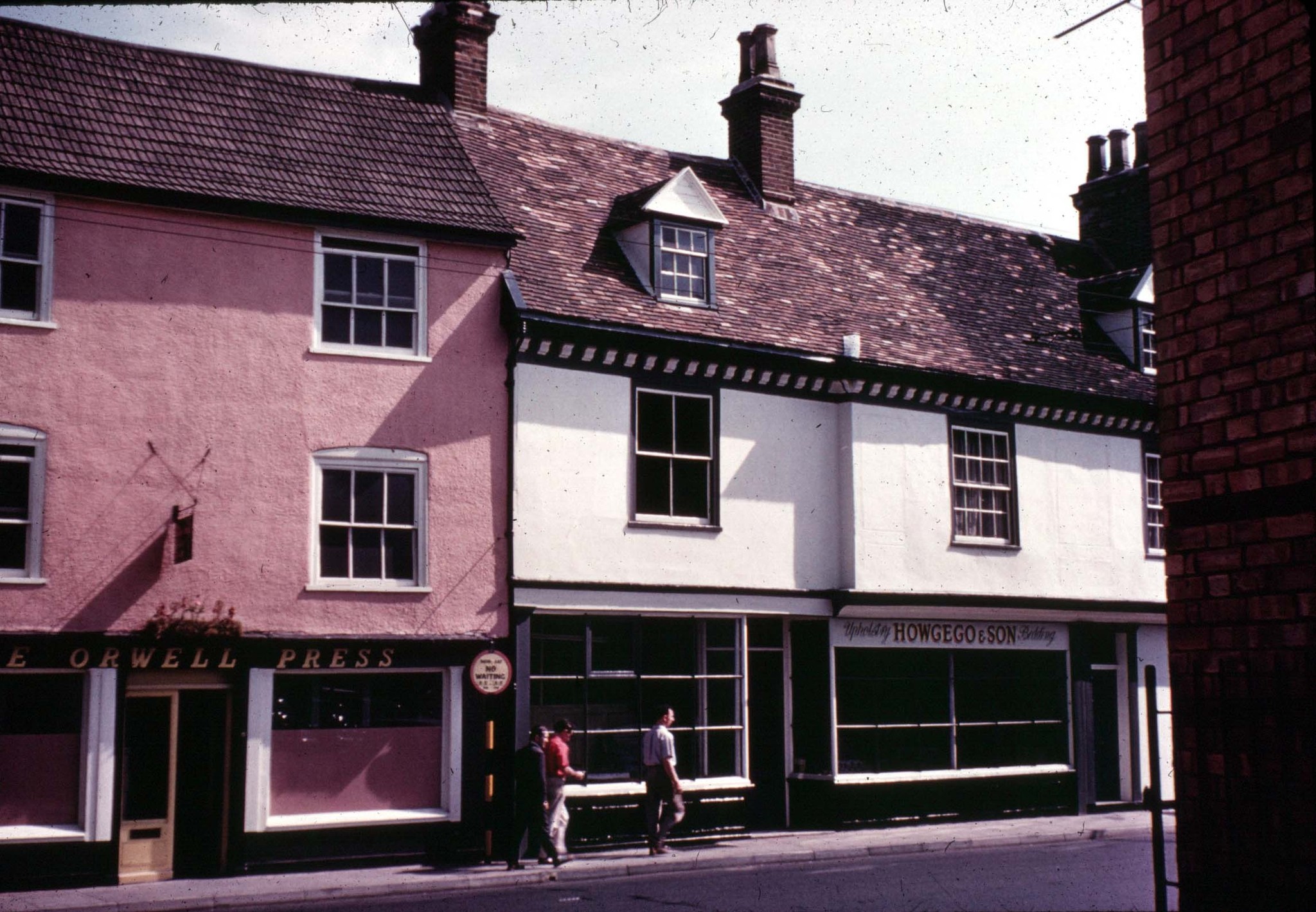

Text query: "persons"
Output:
(537, 717), (587, 864)
(642, 705), (686, 856)
(505, 724), (574, 872)
